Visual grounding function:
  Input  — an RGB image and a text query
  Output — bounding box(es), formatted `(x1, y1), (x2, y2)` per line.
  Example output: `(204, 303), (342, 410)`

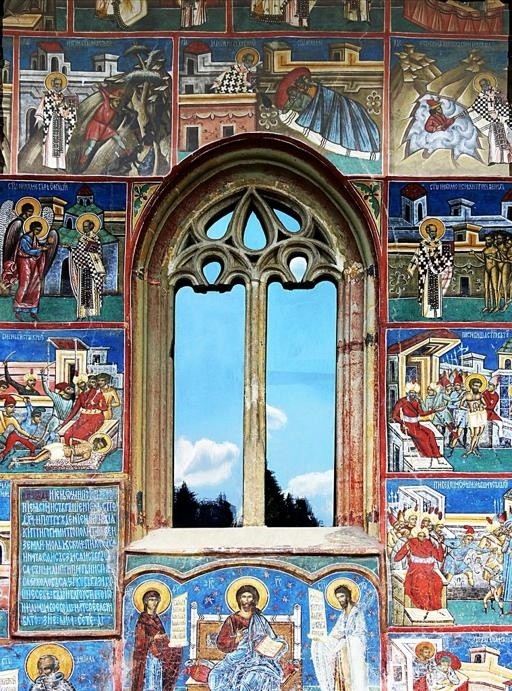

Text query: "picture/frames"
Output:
(8, 478), (126, 636)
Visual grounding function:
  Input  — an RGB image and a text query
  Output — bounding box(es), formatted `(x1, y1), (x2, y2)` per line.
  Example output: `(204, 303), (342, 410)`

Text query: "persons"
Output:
(0, 202), (108, 323)
(387, 504), (511, 623)
(29, 70), (76, 169)
(306, 584), (369, 691)
(469, 232), (512, 314)
(28, 654), (78, 691)
(461, 78), (512, 166)
(408, 224), (453, 319)
(78, 84), (134, 170)
(206, 590), (289, 690)
(411, 641), (435, 675)
(424, 97), (456, 132)
(214, 59), (315, 114)
(128, 592), (184, 691)
(0, 352), (119, 465)
(426, 656), (462, 691)
(394, 375), (498, 465)
